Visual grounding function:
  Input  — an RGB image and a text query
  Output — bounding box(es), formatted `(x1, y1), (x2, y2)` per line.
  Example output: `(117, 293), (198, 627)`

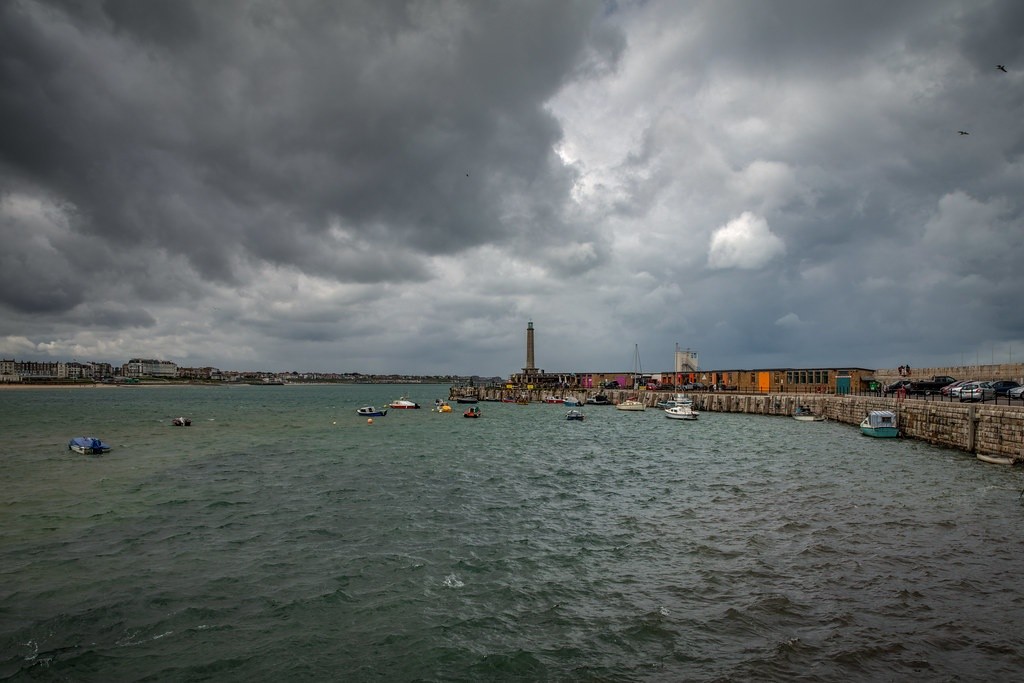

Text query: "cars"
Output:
(603, 380), (620, 389)
(681, 382), (705, 391)
(645, 382), (675, 391)
(882, 380), (913, 394)
(940, 378), (1024, 403)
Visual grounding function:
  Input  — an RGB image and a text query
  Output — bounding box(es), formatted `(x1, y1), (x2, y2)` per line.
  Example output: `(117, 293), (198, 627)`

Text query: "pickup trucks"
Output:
(902, 375), (957, 396)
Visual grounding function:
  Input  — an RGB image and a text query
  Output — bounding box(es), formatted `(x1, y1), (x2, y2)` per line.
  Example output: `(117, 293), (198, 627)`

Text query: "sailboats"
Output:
(616, 343), (648, 411)
(656, 342), (700, 420)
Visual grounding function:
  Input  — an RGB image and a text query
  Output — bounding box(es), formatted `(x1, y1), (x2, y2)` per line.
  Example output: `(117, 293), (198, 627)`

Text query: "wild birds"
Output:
(996, 65), (1007, 73)
(957, 131), (970, 135)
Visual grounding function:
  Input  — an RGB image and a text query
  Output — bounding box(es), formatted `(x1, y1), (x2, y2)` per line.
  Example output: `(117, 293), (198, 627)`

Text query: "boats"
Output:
(976, 453), (1015, 465)
(562, 395), (585, 406)
(173, 417), (192, 426)
(356, 406), (388, 417)
(433, 398), (452, 413)
(791, 404), (825, 422)
(456, 396), (478, 404)
(389, 397), (420, 410)
(545, 394), (565, 404)
(463, 406), (481, 417)
(858, 410), (903, 439)
(67, 436), (111, 456)
(502, 394), (543, 405)
(565, 409), (584, 420)
(587, 394), (612, 405)
